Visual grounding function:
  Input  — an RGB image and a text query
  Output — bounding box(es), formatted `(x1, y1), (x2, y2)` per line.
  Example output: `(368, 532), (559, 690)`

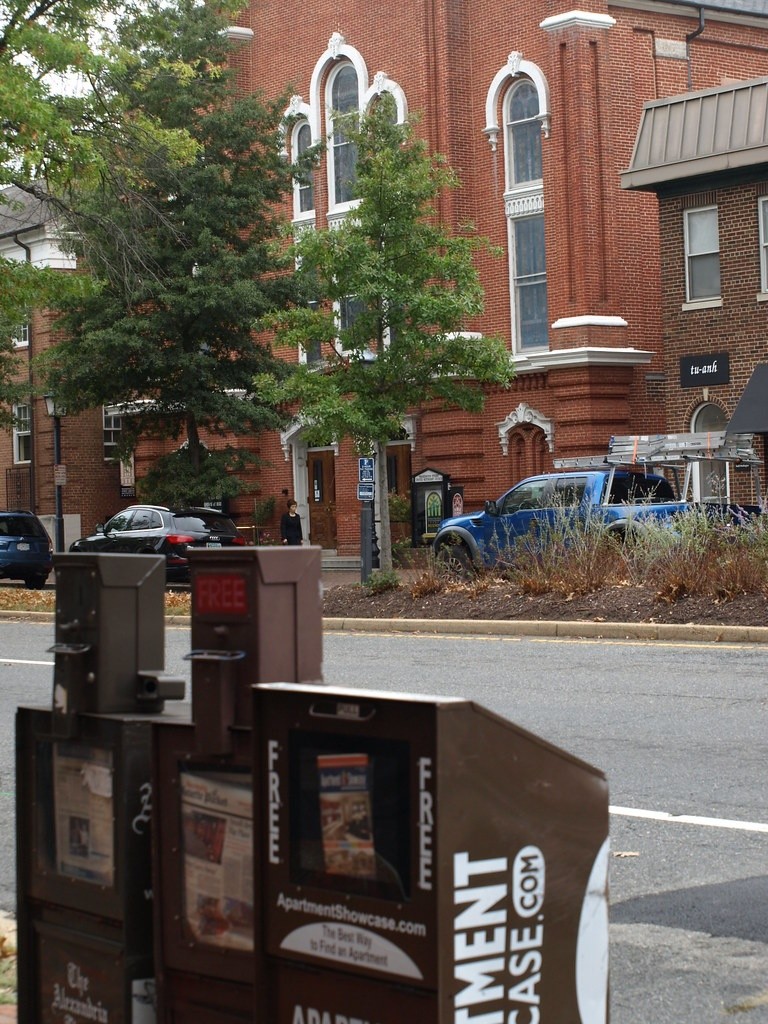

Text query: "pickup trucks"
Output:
(431, 471), (762, 583)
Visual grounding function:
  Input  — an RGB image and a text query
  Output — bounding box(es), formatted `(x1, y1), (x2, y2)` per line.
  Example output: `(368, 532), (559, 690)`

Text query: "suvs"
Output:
(0, 509), (54, 590)
(69, 504), (246, 579)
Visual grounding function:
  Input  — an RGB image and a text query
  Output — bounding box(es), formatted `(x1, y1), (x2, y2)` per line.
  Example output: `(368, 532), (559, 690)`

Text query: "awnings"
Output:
(727, 362), (768, 433)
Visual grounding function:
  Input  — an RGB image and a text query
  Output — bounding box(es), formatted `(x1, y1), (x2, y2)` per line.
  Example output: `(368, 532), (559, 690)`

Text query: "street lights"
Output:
(43, 387), (69, 551)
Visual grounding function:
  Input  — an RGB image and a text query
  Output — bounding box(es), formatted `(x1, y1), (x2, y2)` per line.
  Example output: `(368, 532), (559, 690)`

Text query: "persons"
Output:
(280, 499), (303, 545)
